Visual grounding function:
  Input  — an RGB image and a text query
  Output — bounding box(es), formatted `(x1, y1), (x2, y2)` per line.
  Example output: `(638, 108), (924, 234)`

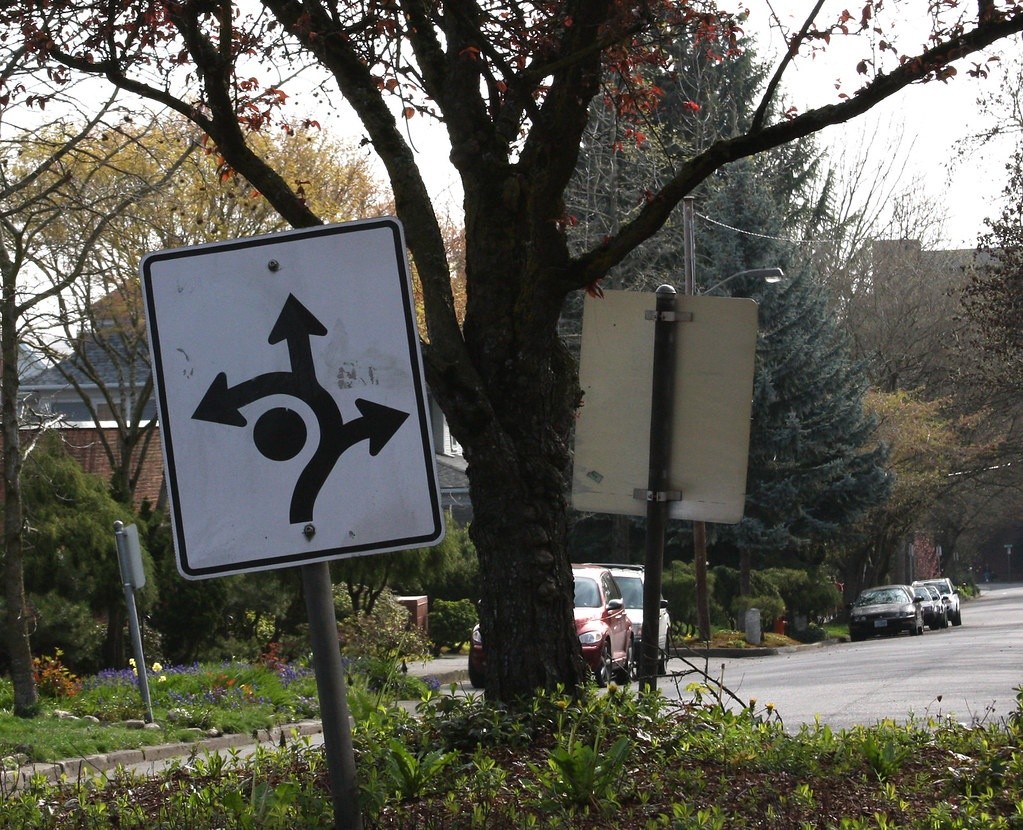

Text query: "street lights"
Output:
(685, 266), (791, 643)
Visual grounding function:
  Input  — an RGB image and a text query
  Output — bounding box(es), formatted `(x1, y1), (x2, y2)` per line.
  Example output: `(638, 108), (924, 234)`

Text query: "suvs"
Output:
(911, 578), (962, 626)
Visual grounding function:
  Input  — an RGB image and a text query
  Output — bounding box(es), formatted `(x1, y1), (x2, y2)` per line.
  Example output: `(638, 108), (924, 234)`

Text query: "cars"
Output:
(925, 584), (949, 628)
(584, 561), (673, 677)
(849, 585), (925, 641)
(468, 564), (634, 688)
(914, 586), (938, 629)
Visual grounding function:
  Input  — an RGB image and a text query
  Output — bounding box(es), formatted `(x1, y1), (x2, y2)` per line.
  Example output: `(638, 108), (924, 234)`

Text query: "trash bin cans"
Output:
(394, 595), (430, 634)
